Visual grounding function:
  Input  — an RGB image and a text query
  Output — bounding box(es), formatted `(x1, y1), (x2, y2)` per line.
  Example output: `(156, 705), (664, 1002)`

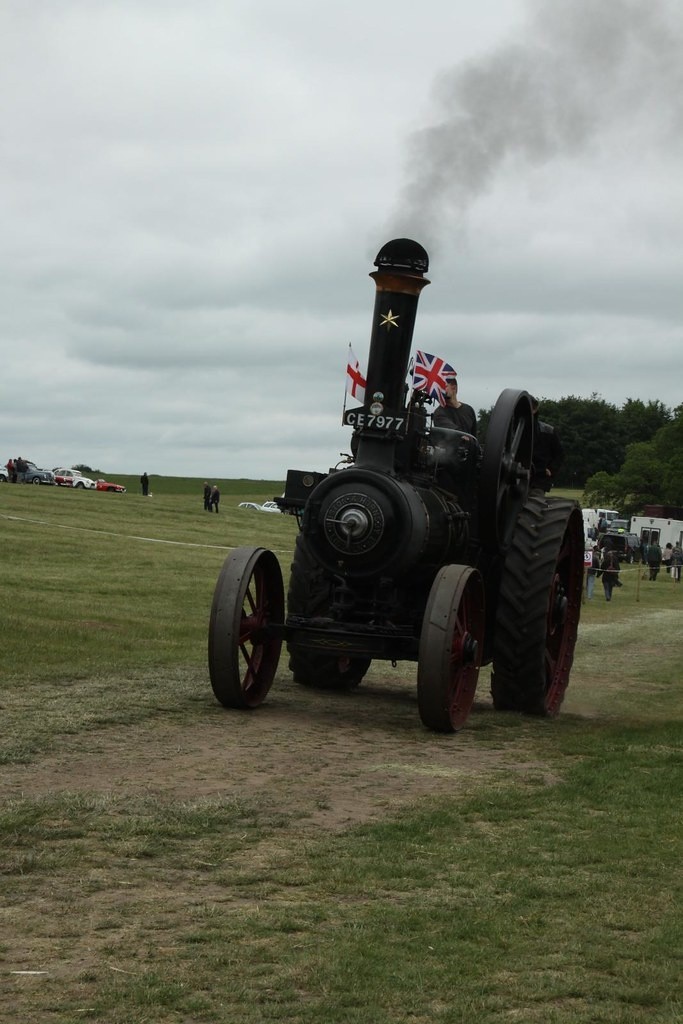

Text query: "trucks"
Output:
(582, 507), (620, 551)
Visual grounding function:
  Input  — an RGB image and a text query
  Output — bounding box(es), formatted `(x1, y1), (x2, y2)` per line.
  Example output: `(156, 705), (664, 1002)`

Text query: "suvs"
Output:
(606, 518), (630, 533)
(596, 527), (641, 564)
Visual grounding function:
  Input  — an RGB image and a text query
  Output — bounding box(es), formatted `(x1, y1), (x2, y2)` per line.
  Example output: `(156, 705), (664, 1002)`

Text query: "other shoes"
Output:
(606, 597), (611, 601)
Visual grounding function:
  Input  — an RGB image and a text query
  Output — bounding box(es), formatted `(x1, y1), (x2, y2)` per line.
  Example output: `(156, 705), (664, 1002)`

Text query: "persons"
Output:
(203, 482), (220, 514)
(640, 539), (683, 583)
(432, 378), (477, 438)
(140, 472), (148, 496)
(6, 457), (26, 485)
(585, 538), (623, 602)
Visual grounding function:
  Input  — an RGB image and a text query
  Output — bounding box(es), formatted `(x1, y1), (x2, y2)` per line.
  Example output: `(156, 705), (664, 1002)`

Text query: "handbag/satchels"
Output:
(605, 566), (616, 576)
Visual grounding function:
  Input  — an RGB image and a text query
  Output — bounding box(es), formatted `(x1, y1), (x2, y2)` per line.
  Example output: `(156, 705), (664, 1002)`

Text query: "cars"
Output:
(6, 458), (127, 493)
(237, 501), (291, 514)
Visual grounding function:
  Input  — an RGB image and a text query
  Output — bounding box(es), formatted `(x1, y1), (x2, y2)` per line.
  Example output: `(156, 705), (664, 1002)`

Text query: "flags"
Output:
(412, 350), (457, 408)
(345, 348), (366, 405)
